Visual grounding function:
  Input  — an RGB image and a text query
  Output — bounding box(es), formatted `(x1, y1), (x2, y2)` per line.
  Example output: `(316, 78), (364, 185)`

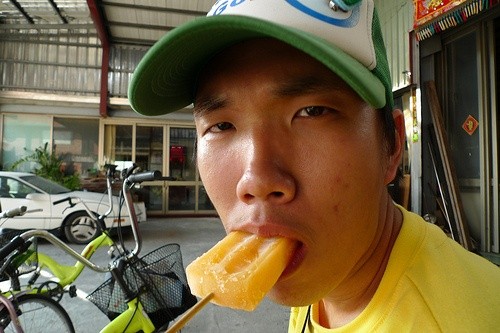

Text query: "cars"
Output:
(0, 167), (147, 245)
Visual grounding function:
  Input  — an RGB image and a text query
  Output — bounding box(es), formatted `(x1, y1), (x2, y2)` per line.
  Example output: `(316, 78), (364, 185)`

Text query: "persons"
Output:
(127, 0), (500, 333)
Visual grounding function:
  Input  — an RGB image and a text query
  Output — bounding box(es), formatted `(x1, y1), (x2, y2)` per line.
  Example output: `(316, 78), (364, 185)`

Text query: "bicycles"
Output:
(0, 167), (170, 333)
(0, 205), (84, 333)
(0, 172), (197, 333)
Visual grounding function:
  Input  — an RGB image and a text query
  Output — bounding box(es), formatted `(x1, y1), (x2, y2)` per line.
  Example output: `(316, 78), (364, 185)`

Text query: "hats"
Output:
(127, 0), (396, 117)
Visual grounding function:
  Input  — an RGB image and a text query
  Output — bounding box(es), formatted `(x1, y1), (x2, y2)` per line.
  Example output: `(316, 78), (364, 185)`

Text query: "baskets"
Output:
(85, 241), (197, 333)
